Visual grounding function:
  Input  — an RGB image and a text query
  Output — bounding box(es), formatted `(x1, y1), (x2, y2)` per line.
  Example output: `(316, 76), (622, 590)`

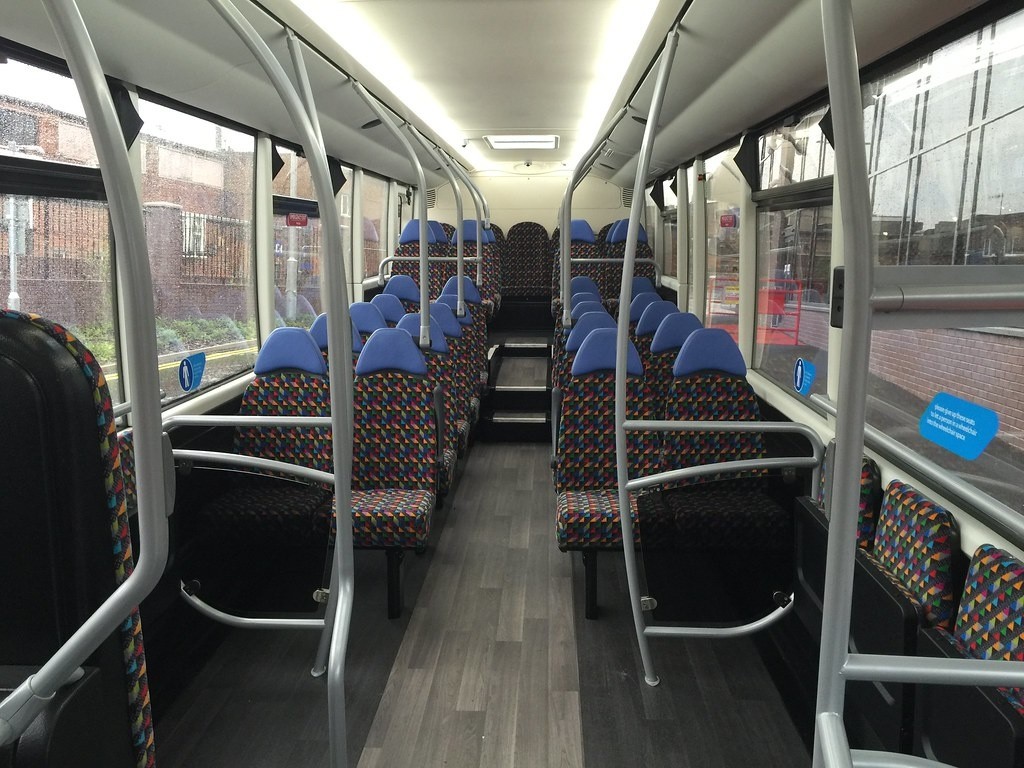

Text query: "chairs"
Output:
(0, 218), (1024, 768)
